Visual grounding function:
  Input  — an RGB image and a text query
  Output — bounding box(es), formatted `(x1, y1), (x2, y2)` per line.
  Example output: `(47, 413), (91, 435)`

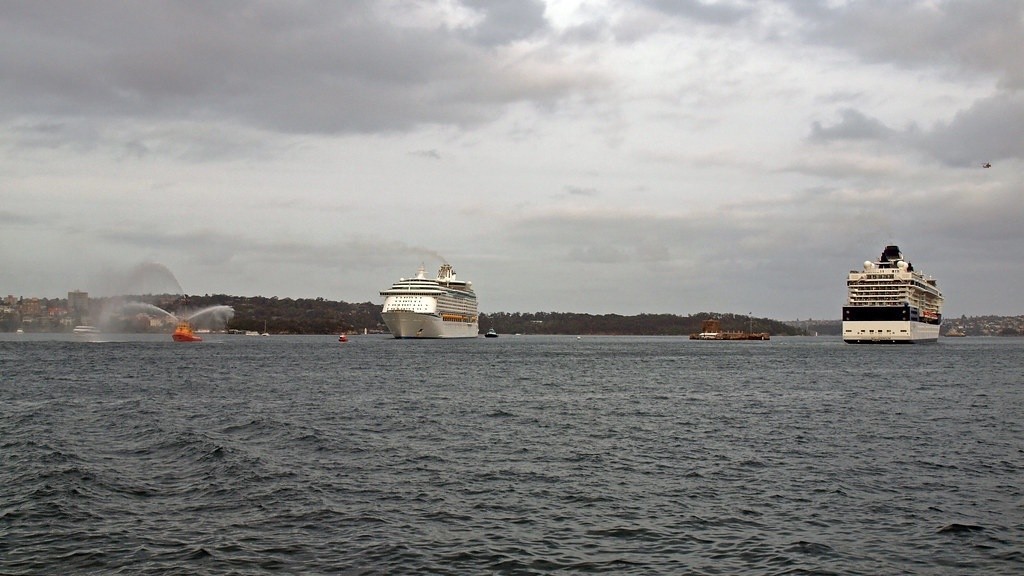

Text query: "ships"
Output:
(379, 262), (479, 340)
(841, 245), (944, 344)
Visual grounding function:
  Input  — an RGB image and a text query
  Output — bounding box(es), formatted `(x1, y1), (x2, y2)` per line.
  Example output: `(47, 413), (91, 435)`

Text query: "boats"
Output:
(17, 328), (24, 334)
(516, 333), (522, 336)
(246, 330), (259, 336)
(944, 327), (966, 337)
(338, 332), (349, 342)
(689, 311), (770, 341)
(73, 324), (100, 335)
(484, 314), (499, 338)
(262, 320), (271, 337)
(171, 327), (203, 342)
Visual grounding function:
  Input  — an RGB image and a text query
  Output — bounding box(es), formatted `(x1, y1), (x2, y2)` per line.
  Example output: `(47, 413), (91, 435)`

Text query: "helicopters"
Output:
(981, 162), (991, 168)
